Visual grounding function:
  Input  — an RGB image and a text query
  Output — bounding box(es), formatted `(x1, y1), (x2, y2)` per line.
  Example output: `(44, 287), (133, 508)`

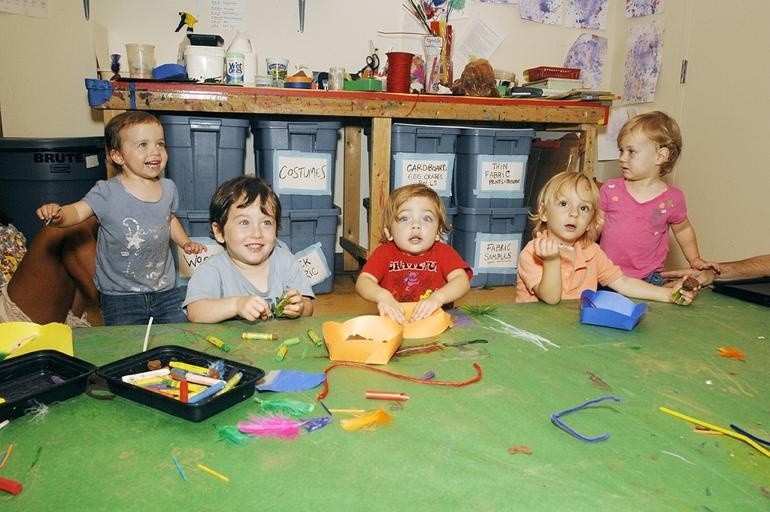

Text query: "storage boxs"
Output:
(458, 124), (535, 212)
(168, 211), (223, 271)
(157, 115), (251, 214)
(455, 205), (534, 289)
(390, 123), (460, 210)
(250, 122), (343, 203)
(276, 204), (343, 295)
(362, 197), (457, 259)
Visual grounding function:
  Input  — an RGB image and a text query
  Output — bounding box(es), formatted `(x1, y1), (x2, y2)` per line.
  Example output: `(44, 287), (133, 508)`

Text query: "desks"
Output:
(0, 280), (770, 512)
(84, 76), (613, 282)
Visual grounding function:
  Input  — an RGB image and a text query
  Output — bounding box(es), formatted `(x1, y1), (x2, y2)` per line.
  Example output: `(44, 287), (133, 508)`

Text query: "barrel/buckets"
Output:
(183, 45), (224, 85)
(224, 30), (257, 85)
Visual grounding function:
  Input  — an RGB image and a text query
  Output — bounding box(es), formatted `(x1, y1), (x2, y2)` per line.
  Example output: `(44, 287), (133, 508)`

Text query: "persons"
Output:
(181, 175), (317, 324)
(36, 110), (207, 327)
(0, 215), (100, 328)
(515, 171), (701, 306)
(588, 111), (721, 292)
(659, 254), (770, 289)
(355, 183), (474, 325)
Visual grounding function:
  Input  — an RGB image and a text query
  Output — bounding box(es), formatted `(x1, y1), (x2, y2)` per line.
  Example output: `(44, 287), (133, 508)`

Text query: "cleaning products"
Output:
(174, 10), (198, 66)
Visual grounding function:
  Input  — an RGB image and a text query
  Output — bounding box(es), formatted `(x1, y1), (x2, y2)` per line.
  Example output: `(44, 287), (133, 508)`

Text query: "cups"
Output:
(385, 51), (416, 94)
(127, 43), (157, 78)
(228, 52), (246, 84)
(267, 57), (290, 87)
(329, 67), (345, 91)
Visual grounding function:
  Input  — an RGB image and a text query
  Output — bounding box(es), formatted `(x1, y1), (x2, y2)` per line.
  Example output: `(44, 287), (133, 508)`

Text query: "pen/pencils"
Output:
(0, 416), (15, 429)
(44, 206), (62, 226)
(328, 409), (365, 413)
(321, 401), (333, 416)
(50, 375), (65, 385)
(241, 332), (279, 341)
(206, 335), (231, 352)
(276, 338), (300, 362)
(121, 361), (244, 404)
(197, 464), (229, 482)
(170, 453), (188, 482)
(307, 329), (323, 347)
